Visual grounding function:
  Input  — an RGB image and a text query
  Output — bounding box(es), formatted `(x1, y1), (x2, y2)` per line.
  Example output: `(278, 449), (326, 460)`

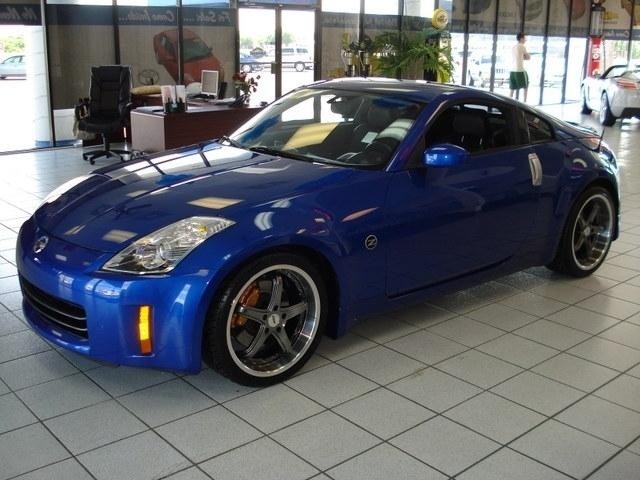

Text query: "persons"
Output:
(507, 32), (532, 102)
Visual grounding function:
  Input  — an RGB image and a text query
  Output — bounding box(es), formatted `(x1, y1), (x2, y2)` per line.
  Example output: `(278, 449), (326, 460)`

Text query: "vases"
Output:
(232, 72), (260, 94)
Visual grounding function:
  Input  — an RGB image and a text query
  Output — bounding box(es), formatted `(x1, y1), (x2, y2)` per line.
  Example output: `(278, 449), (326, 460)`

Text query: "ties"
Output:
(201, 70), (219, 99)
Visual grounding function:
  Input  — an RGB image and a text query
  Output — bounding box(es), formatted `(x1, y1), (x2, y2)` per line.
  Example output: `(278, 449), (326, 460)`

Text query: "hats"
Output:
(0, 55), (26, 79)
(448, 41), (565, 90)
(152, 27), (224, 85)
(240, 46), (313, 72)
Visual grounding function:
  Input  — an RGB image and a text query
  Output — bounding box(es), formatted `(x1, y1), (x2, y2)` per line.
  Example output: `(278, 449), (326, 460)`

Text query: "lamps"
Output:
(74, 64), (144, 165)
(347, 103), (399, 164)
(433, 108), (490, 154)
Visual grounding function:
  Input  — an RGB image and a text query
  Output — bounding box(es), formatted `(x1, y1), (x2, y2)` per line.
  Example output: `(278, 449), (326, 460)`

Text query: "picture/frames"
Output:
(130, 93), (267, 159)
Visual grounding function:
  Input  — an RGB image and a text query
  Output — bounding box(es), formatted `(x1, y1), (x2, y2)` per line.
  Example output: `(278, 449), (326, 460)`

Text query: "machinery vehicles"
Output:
(580, 63), (640, 125)
(14, 77), (619, 386)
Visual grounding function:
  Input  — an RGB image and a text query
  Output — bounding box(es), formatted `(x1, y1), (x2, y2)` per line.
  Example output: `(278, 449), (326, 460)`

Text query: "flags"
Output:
(242, 94), (250, 104)
